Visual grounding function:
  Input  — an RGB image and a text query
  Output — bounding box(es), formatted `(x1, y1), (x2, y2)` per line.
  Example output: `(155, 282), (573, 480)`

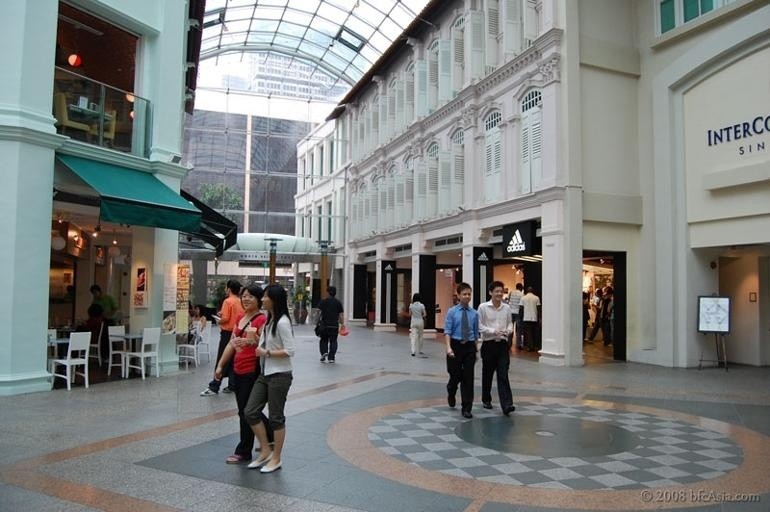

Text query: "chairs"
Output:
(47, 328), (58, 380)
(54, 92), (90, 137)
(175, 318), (213, 371)
(84, 109), (117, 148)
(48, 331), (93, 392)
(77, 321), (105, 368)
(107, 325), (133, 378)
(122, 327), (162, 380)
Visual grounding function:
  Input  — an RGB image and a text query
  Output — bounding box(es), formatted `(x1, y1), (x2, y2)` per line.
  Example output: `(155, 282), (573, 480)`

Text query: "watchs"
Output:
(265, 349), (271, 359)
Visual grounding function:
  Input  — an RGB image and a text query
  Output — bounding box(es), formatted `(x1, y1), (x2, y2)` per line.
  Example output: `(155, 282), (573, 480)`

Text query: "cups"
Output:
(230, 336), (244, 353)
(245, 326), (257, 342)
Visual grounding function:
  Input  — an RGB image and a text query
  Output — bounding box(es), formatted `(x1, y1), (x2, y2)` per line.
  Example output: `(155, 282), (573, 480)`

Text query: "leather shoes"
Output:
(448, 394), (455, 407)
(503, 405), (515, 415)
(461, 407), (472, 418)
(248, 451), (273, 470)
(260, 458), (282, 472)
(483, 402), (492, 409)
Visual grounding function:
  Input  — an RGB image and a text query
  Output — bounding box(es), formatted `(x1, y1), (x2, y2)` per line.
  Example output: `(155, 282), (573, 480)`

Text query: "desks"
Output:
(50, 336), (83, 368)
(109, 331), (144, 379)
(63, 104), (112, 127)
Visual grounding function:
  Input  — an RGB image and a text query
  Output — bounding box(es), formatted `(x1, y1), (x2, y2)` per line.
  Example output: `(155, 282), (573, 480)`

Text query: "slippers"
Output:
(226, 454), (242, 463)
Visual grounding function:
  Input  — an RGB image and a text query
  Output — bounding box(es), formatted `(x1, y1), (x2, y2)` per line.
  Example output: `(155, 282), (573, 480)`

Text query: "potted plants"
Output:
(289, 282), (312, 324)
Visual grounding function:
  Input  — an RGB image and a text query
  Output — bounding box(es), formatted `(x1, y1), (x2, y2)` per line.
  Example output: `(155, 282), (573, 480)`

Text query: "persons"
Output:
(200, 279), (244, 396)
(89, 286), (120, 322)
(63, 284), (76, 300)
(443, 283), (482, 419)
(475, 281), (519, 414)
(407, 292), (426, 356)
(517, 286), (542, 352)
(315, 285), (344, 363)
(187, 304), (206, 335)
(243, 283), (296, 473)
(505, 282), (525, 351)
(72, 304), (107, 343)
(583, 284), (616, 348)
(213, 286), (276, 464)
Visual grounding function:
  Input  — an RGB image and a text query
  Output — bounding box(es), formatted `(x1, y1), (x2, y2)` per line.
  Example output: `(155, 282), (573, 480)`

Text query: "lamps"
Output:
(52, 213), (126, 261)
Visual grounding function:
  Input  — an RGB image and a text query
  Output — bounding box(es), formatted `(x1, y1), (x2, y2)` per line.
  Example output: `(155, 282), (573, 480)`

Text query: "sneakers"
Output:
(320, 353), (329, 361)
(223, 386), (230, 392)
(200, 387), (218, 396)
(329, 359), (335, 363)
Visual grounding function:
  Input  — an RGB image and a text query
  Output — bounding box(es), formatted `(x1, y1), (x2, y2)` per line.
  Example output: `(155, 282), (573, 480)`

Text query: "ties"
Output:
(462, 308), (469, 342)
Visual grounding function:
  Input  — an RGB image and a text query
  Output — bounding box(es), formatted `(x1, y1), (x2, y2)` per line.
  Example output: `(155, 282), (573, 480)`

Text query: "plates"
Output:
(162, 312), (176, 335)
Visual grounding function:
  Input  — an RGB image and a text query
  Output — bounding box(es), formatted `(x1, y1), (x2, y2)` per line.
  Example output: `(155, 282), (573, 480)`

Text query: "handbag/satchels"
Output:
(314, 318), (322, 337)
